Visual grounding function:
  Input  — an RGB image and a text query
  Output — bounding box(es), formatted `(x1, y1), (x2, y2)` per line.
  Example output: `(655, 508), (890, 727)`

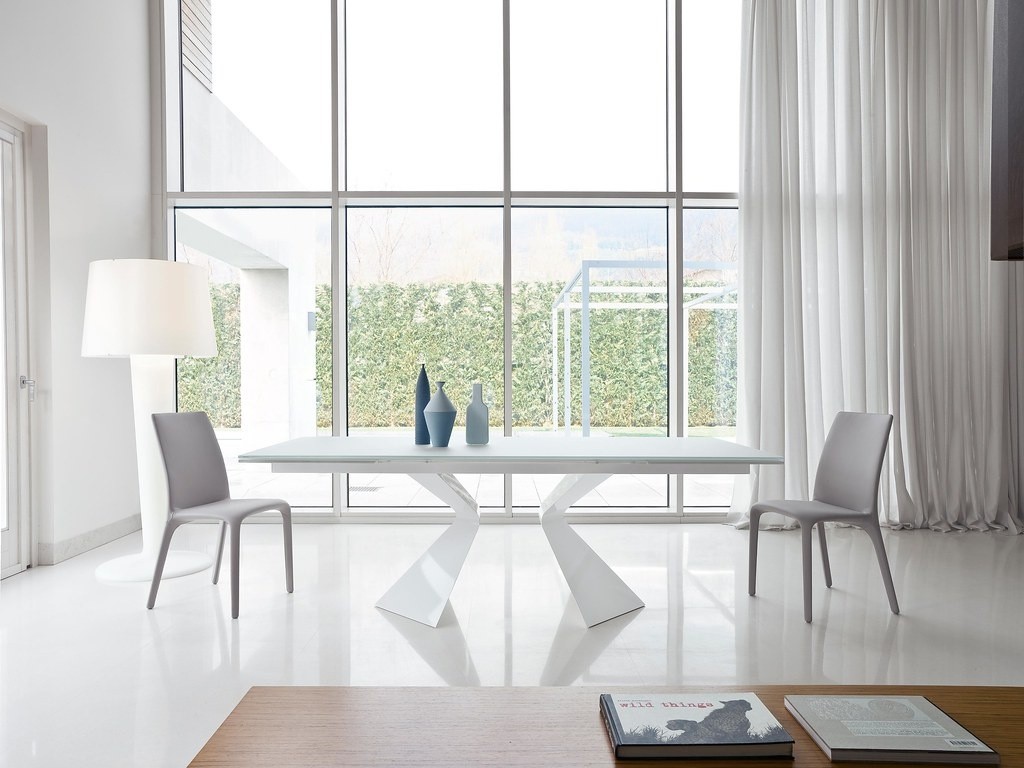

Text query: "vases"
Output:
(415, 364), (430, 444)
(422, 381), (457, 448)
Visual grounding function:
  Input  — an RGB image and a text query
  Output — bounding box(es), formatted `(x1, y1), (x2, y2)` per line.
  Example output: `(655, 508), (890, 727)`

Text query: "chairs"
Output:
(748, 412), (899, 622)
(145, 412), (294, 619)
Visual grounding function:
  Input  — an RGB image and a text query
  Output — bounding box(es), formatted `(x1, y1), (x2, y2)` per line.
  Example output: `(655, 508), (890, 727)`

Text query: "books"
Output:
(599, 691), (796, 761)
(784, 694), (1001, 766)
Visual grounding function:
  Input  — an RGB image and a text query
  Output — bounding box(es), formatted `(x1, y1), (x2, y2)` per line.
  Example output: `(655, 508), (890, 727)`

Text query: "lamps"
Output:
(84, 260), (217, 583)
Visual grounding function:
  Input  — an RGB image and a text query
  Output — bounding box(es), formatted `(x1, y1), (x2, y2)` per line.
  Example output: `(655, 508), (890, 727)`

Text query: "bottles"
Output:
(466, 383), (489, 444)
(415, 362), (457, 448)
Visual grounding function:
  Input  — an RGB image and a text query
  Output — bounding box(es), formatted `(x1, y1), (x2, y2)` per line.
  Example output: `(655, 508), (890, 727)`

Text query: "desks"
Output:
(184, 685), (1024, 768)
(238, 436), (783, 629)
